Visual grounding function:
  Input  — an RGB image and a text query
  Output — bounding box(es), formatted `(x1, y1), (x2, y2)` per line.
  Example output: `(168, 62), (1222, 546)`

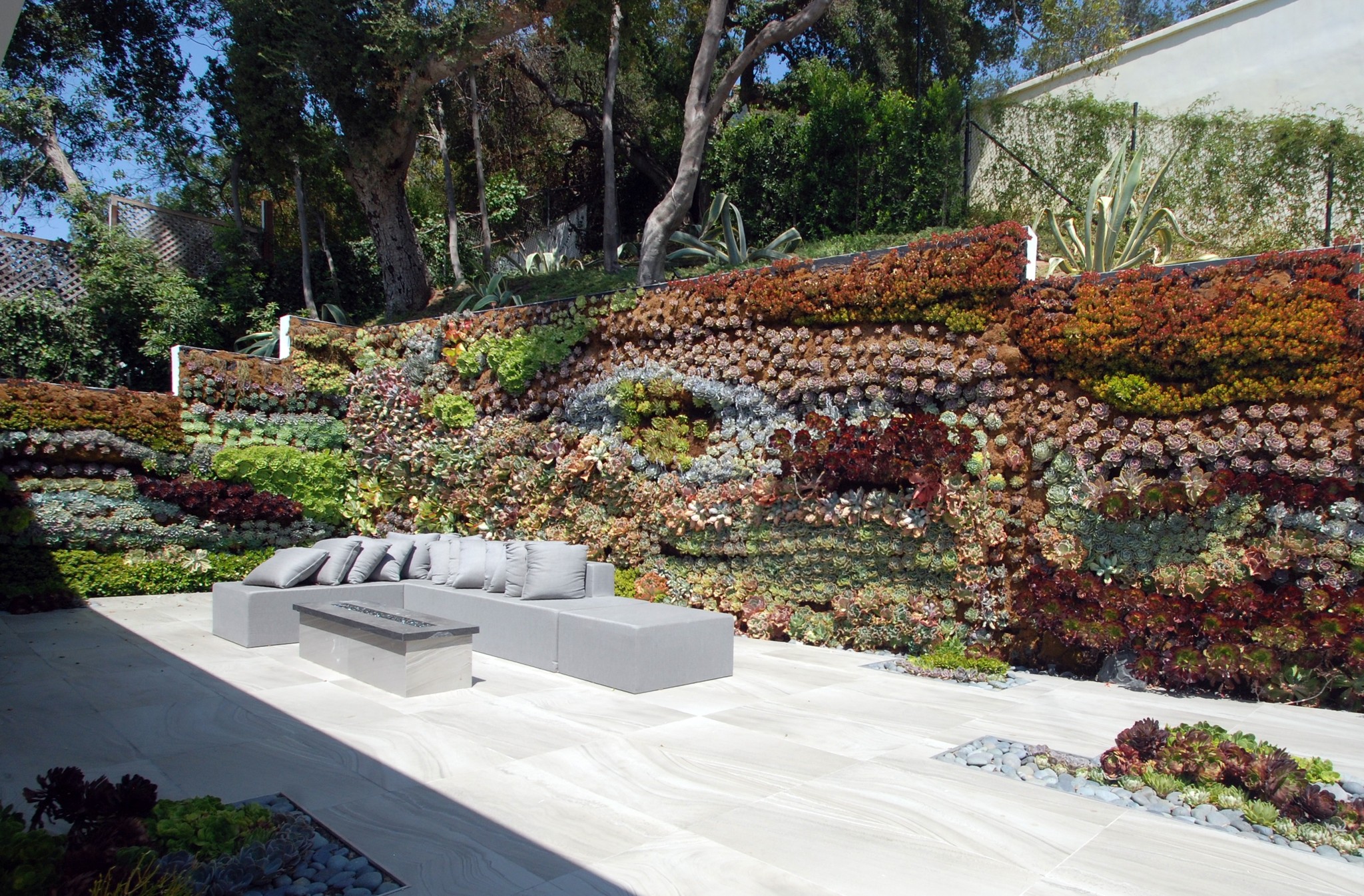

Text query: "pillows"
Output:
(503, 541), (569, 597)
(486, 542), (508, 593)
(342, 535), (392, 584)
(443, 537), (474, 586)
(482, 540), (504, 590)
(308, 538), (363, 586)
(426, 533), (459, 579)
(386, 532), (441, 579)
(521, 540), (589, 599)
(452, 539), (487, 589)
(242, 547), (330, 589)
(425, 534), (483, 584)
(369, 538), (415, 582)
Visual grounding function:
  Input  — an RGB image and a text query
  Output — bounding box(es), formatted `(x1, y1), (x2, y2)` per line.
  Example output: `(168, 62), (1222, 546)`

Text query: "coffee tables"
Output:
(293, 599), (479, 698)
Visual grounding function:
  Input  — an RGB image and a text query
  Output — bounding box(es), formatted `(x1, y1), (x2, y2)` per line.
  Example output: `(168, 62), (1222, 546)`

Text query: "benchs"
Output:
(212, 556), (734, 694)
